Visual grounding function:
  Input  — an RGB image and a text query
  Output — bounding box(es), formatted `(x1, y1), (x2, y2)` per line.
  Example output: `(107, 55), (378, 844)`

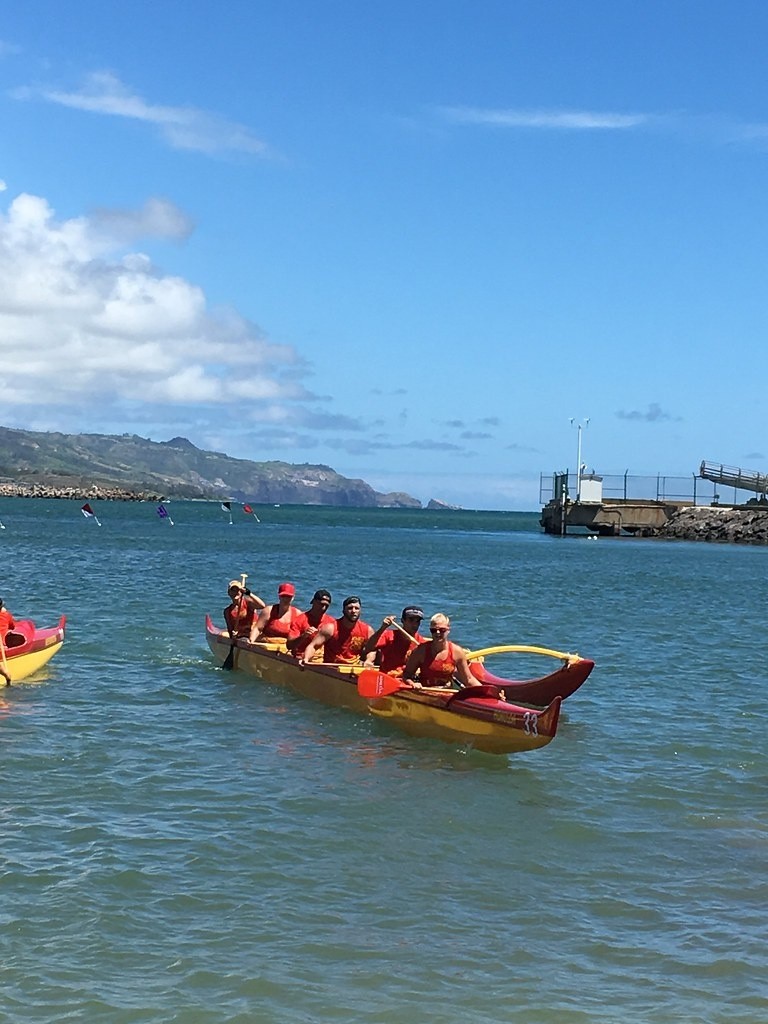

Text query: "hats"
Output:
(228, 580), (242, 593)
(402, 606), (424, 620)
(310, 589), (331, 604)
(278, 583), (295, 597)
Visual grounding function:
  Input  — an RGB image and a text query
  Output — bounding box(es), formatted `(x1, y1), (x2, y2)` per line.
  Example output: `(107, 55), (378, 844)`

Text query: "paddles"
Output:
(355, 668), (459, 699)
(301, 659), (362, 668)
(248, 640), (270, 646)
(222, 573), (249, 671)
(0, 635), (12, 686)
(390, 615), (420, 646)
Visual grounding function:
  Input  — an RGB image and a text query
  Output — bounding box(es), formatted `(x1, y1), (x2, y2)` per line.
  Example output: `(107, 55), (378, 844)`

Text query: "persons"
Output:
(403, 614), (507, 702)
(364, 606), (427, 684)
(0, 599), (16, 649)
(286, 589), (336, 663)
(298, 596), (375, 671)
(246, 584), (304, 649)
(222, 580), (265, 639)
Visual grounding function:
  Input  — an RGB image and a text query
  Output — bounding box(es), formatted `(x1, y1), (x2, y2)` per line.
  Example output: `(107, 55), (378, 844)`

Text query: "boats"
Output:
(0, 615), (66, 687)
(205, 616), (595, 754)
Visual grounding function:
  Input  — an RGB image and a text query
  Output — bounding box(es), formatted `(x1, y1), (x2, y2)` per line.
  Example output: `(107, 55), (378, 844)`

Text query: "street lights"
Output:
(570, 418), (591, 502)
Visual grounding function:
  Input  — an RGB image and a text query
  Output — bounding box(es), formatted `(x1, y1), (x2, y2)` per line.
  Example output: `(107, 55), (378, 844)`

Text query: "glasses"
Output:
(429, 627), (448, 633)
